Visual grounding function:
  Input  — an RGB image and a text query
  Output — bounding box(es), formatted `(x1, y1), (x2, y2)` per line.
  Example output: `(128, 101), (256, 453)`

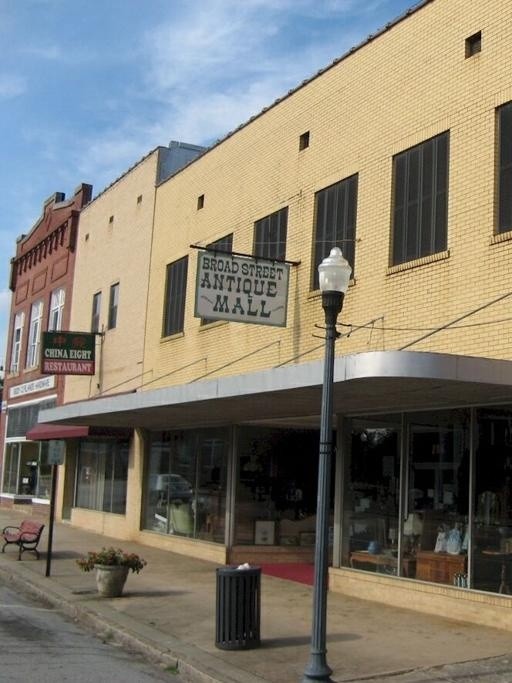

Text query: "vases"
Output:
(94, 561), (129, 598)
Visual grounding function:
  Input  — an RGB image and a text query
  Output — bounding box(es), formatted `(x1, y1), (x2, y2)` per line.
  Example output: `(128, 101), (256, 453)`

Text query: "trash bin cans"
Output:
(215, 564), (262, 650)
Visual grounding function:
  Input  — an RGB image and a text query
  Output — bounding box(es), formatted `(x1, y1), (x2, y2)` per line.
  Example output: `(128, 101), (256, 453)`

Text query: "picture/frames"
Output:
(298, 530), (316, 546)
(252, 518), (277, 546)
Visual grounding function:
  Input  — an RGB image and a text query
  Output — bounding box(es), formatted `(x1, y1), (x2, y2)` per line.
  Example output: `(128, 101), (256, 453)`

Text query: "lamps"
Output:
(404, 512), (422, 551)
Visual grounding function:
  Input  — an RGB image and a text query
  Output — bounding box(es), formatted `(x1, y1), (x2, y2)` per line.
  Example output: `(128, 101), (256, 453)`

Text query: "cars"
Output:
(150, 474), (193, 505)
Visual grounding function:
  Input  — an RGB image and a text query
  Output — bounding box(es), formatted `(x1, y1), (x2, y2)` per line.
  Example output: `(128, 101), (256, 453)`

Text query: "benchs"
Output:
(0, 519), (45, 562)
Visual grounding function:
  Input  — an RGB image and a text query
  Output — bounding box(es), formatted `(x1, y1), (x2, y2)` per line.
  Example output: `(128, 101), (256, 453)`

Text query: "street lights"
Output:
(302, 247), (352, 683)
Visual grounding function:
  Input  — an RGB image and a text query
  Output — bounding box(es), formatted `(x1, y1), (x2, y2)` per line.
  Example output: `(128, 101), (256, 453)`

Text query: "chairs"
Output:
(166, 503), (203, 536)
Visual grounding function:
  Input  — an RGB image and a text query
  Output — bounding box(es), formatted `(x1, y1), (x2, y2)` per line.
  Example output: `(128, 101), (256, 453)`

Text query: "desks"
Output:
(350, 551), (414, 578)
(482, 549), (512, 596)
(414, 551), (466, 586)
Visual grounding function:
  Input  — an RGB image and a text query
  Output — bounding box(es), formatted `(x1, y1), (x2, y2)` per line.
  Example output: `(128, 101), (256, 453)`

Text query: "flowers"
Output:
(76, 545), (147, 576)
